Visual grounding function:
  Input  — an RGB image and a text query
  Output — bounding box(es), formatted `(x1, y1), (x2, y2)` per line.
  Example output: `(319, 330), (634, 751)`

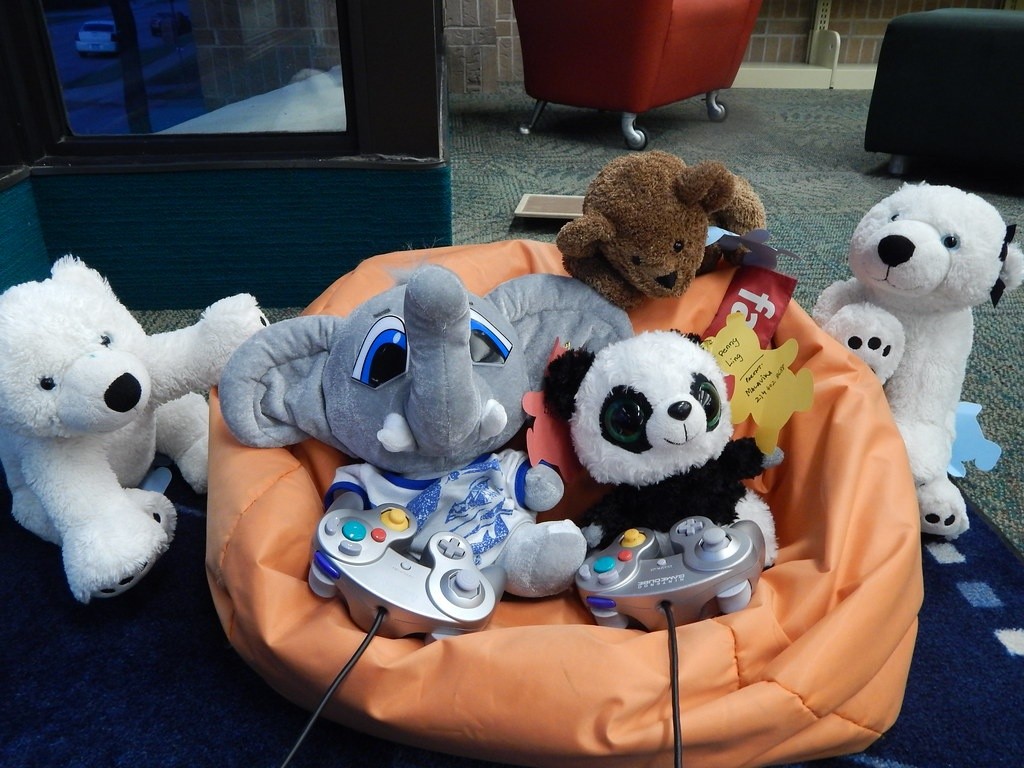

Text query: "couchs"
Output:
(512, 0), (766, 151)
(863, 7), (1024, 176)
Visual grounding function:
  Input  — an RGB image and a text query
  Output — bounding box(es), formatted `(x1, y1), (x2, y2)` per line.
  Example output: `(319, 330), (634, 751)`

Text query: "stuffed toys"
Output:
(555, 150), (768, 309)
(544, 329), (777, 569)
(0, 255), (271, 605)
(219, 262), (633, 599)
(811, 180), (1024, 538)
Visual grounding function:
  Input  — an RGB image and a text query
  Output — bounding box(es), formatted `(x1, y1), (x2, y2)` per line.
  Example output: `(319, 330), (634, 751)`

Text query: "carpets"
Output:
(131, 81), (1024, 554)
(0, 449), (1024, 768)
